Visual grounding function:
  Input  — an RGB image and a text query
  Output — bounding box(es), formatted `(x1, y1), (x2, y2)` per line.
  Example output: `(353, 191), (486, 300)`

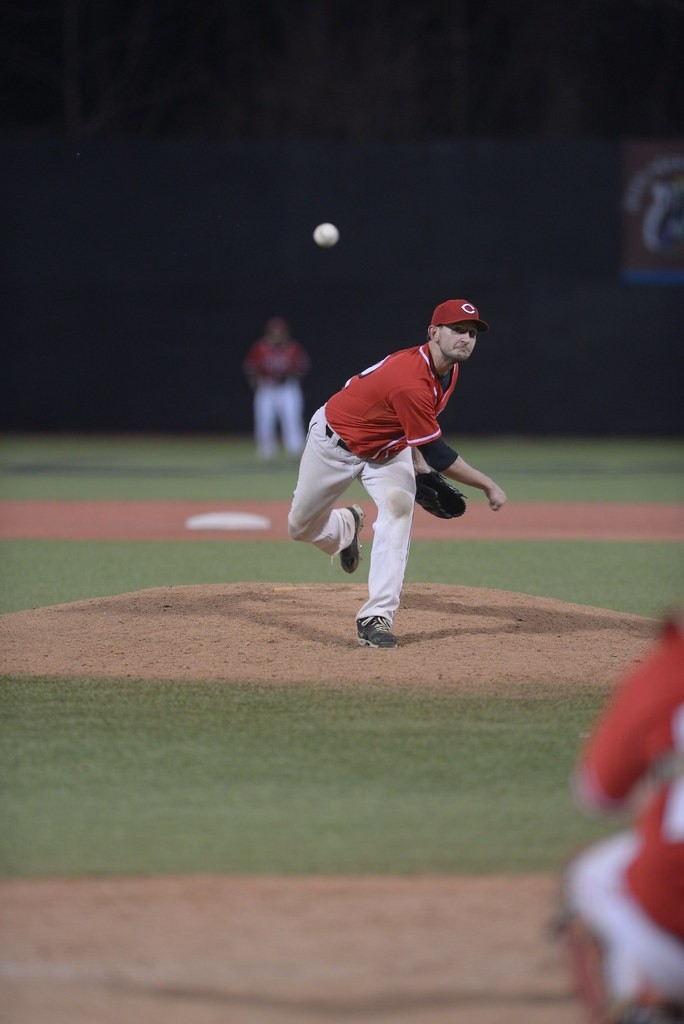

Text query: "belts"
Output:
(326, 425), (351, 452)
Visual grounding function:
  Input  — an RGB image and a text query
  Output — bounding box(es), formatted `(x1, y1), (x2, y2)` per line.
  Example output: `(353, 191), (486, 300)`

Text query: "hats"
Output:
(430, 299), (488, 332)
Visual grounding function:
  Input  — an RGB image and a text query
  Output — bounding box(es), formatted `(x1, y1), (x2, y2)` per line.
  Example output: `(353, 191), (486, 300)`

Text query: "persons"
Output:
(550, 604), (684, 1023)
(288, 298), (506, 650)
(241, 317), (306, 455)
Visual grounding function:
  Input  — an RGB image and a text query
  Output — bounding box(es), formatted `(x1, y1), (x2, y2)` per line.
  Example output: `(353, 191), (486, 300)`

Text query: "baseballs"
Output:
(312, 222), (340, 250)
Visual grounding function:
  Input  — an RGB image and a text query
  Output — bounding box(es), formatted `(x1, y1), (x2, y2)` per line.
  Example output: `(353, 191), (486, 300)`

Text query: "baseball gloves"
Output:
(413, 469), (467, 521)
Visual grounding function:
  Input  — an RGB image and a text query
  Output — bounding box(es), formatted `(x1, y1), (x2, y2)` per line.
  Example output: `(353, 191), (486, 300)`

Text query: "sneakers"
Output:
(357, 617), (401, 649)
(340, 503), (364, 574)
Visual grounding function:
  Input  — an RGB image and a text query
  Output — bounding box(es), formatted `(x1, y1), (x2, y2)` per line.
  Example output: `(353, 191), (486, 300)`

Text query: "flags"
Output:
(621, 135), (684, 283)
